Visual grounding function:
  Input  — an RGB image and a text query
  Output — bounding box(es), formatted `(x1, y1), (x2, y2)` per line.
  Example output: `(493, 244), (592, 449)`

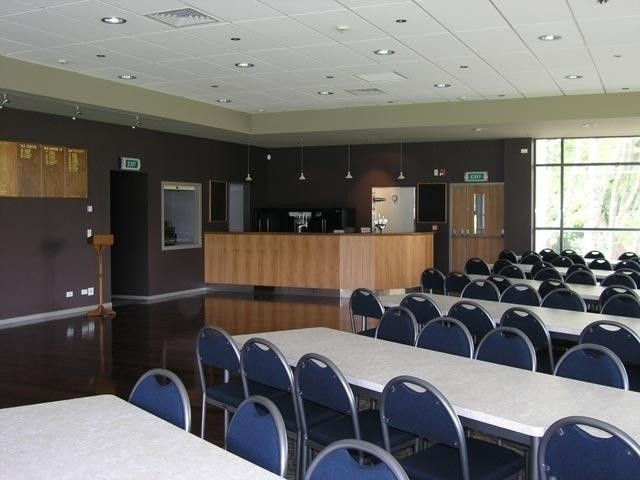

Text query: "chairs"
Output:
(399, 293), (443, 330)
(414, 317), (474, 360)
(195, 325), (285, 451)
(294, 352), (423, 480)
(375, 306), (418, 346)
(443, 270), (472, 296)
(539, 288), (587, 312)
(240, 337), (347, 480)
(349, 288), (385, 334)
(600, 293), (640, 319)
(421, 268), (446, 295)
(552, 343), (629, 392)
(460, 278), (501, 302)
(578, 320), (640, 365)
(498, 283), (542, 307)
(223, 395), (287, 478)
(129, 369), (192, 433)
(475, 327), (537, 372)
(379, 375), (526, 480)
(447, 300), (497, 352)
(464, 246), (640, 289)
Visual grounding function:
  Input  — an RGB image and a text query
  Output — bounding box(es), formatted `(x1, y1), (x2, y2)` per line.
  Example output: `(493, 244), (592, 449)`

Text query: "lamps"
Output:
(245, 146), (253, 181)
(397, 136), (405, 179)
(345, 145), (353, 178)
(345, 171), (353, 179)
(298, 144), (306, 180)
(245, 173), (253, 181)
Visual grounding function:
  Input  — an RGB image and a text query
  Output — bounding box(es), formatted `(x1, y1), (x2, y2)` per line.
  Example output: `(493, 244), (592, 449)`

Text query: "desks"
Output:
(362, 292), (640, 343)
(481, 274), (640, 306)
(0, 393), (288, 480)
(305, 439), (409, 480)
(538, 417), (640, 480)
(499, 307), (557, 376)
